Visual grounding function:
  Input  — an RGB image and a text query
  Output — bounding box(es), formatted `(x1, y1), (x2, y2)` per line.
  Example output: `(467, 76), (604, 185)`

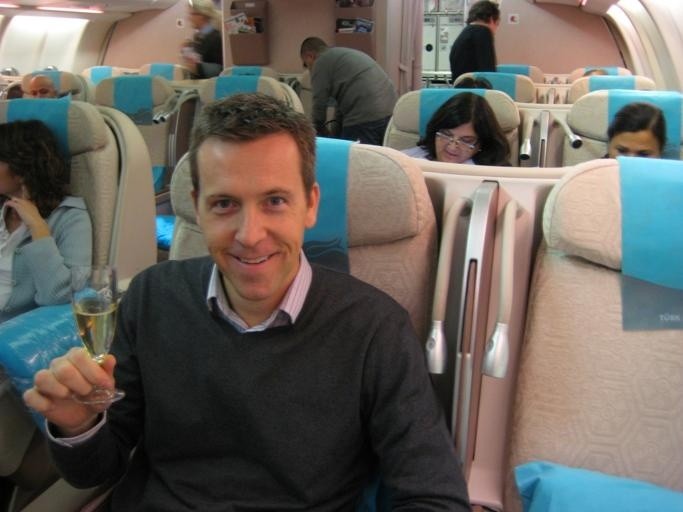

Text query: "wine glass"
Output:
(67, 264), (125, 406)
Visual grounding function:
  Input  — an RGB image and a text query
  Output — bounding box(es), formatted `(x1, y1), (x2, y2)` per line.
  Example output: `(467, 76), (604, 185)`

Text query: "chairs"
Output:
(504, 155), (682, 508)
(170, 135), (437, 349)
(2, 99), (159, 510)
(2, 67), (682, 162)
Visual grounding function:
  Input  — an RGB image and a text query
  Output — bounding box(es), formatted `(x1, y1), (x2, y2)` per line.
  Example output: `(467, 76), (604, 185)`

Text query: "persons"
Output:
(177, 1), (224, 79)
(397, 91), (517, 168)
(21, 92), (473, 512)
(447, 1), (501, 85)
(582, 68), (608, 77)
(298, 37), (397, 148)
(454, 75), (492, 90)
(595, 102), (666, 160)
(28, 74), (58, 99)
(0, 118), (95, 322)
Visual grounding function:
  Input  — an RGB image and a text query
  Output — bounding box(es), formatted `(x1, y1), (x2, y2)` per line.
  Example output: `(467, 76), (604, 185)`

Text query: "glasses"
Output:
(435, 129), (479, 152)
